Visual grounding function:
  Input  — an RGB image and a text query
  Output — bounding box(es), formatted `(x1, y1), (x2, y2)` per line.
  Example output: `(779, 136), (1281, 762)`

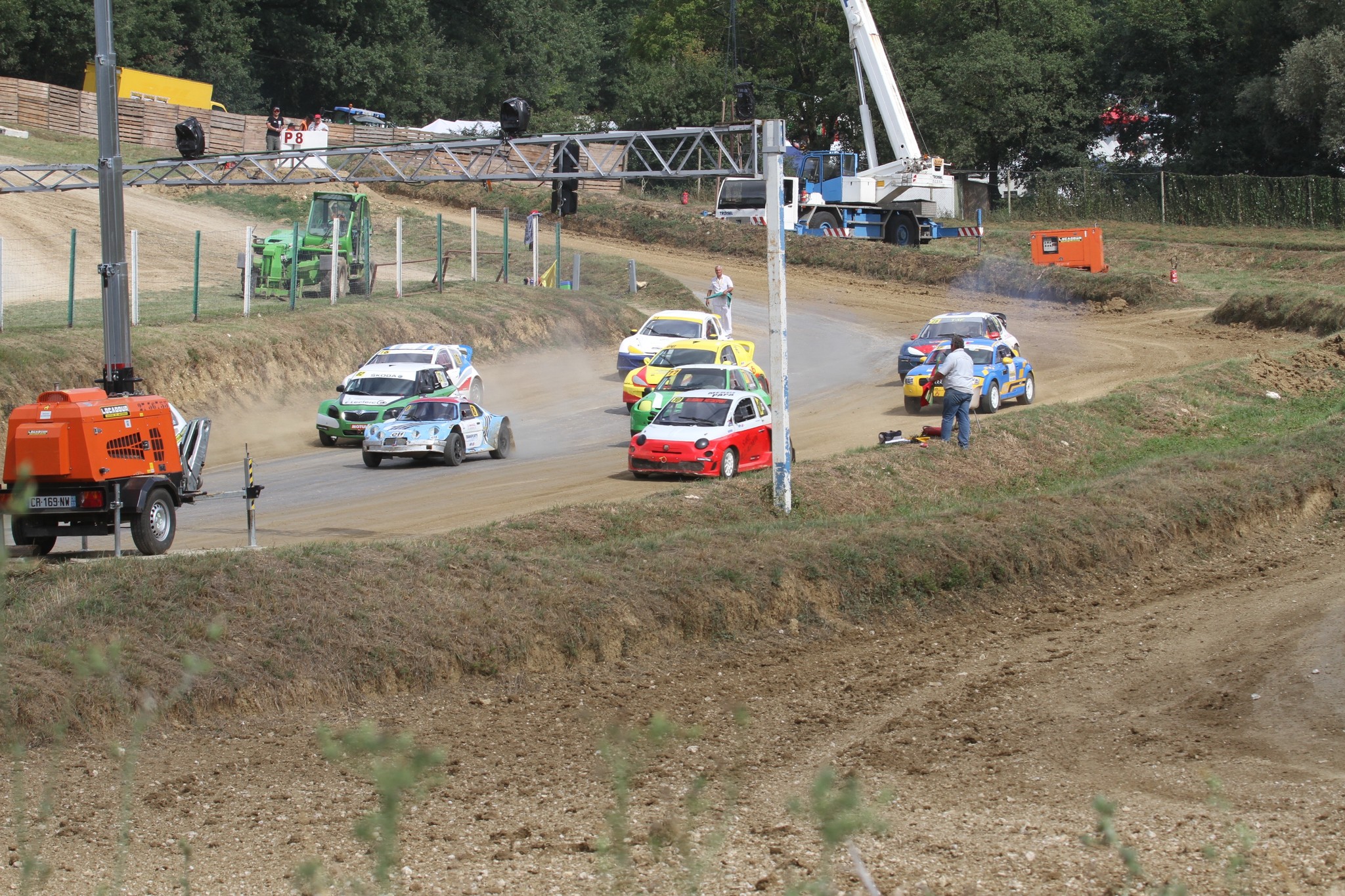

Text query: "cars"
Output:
(898, 311), (1023, 385)
(316, 363), (463, 447)
(627, 389), (797, 480)
(358, 342), (484, 404)
(623, 340), (770, 412)
(362, 396), (517, 469)
(903, 337), (1035, 414)
(617, 309), (737, 381)
(630, 364), (773, 443)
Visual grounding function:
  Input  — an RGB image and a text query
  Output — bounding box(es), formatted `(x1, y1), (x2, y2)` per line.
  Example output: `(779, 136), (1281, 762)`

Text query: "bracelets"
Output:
(276, 128), (278, 132)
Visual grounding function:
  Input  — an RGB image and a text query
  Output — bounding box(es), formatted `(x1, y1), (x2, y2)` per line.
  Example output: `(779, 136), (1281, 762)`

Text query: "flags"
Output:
(540, 259), (557, 288)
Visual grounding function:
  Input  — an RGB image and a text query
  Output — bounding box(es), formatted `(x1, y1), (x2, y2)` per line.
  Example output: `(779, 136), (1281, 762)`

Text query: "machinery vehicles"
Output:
(714, 0), (983, 256)
(1, 0), (223, 557)
(237, 181), (379, 300)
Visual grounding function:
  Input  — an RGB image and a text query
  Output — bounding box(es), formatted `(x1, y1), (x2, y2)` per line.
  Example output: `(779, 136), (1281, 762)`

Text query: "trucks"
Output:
(83, 60), (228, 114)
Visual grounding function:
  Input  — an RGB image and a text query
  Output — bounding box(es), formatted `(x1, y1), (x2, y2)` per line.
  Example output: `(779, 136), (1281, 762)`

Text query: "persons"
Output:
(266, 107), (284, 154)
(286, 123), (297, 131)
(927, 336), (974, 453)
(329, 202), (347, 222)
(706, 265), (734, 338)
(308, 114), (329, 131)
(299, 114), (315, 131)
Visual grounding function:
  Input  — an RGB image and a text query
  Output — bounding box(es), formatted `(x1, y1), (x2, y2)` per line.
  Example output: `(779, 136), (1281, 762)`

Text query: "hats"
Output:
(272, 106), (280, 111)
(314, 114), (321, 119)
(288, 123), (296, 126)
(308, 113), (315, 122)
